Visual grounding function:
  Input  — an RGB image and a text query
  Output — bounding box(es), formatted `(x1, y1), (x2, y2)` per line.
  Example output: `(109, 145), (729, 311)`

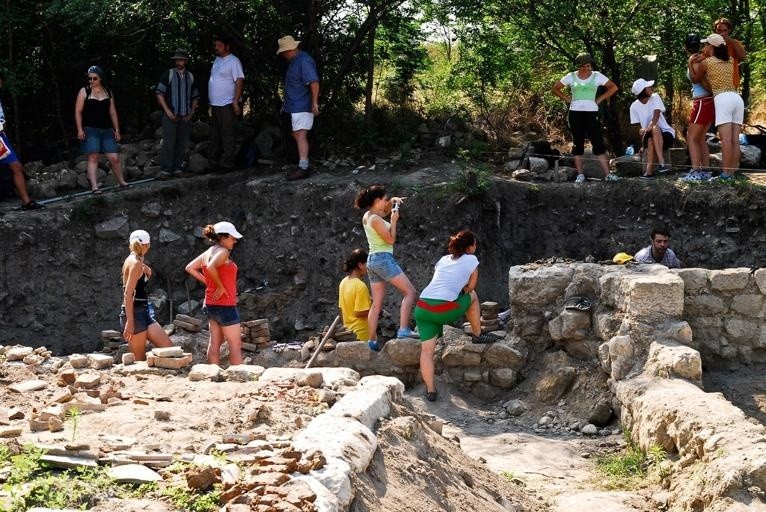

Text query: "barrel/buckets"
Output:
(119, 184), (137, 188)
(92, 190), (103, 195)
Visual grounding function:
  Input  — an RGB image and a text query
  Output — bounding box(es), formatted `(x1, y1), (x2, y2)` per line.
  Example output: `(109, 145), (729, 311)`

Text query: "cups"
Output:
(87, 76), (97, 81)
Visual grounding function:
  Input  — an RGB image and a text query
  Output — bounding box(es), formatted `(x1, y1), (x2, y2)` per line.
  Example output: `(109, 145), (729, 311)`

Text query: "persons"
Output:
(275, 34), (320, 181)
(686, 33), (746, 182)
(551, 51), (623, 184)
(629, 77), (676, 179)
(713, 16), (747, 159)
(155, 48), (201, 174)
(118, 228), (175, 361)
(0, 103), (45, 212)
(337, 248), (378, 342)
(354, 183), (423, 353)
(413, 228), (499, 403)
(678, 32), (714, 183)
(74, 65), (136, 195)
(631, 225), (682, 269)
(183, 221), (243, 367)
(201, 34), (245, 175)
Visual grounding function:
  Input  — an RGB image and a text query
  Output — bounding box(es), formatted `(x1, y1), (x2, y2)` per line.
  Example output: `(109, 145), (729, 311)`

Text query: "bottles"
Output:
(88, 65), (103, 74)
(213, 221), (244, 239)
(129, 230), (150, 245)
(700, 33), (727, 48)
(631, 78), (655, 96)
(276, 35), (302, 55)
(612, 252), (637, 265)
(573, 52), (591, 69)
(170, 49), (190, 60)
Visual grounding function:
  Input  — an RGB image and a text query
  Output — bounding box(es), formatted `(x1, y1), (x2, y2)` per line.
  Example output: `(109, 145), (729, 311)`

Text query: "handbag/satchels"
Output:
(113, 127), (119, 132)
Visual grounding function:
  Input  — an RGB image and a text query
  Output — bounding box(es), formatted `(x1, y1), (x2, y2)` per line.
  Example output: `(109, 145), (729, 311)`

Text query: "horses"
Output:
(395, 203), (400, 210)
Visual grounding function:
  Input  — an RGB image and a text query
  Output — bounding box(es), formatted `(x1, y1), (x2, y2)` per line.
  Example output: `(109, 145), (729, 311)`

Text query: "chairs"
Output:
(172, 169), (185, 177)
(639, 174), (654, 180)
(680, 169), (702, 180)
(471, 329), (498, 344)
(711, 173), (732, 180)
(425, 386), (438, 402)
(287, 167), (310, 180)
(21, 199), (47, 211)
(700, 170), (713, 180)
(605, 174), (623, 181)
(656, 164), (670, 173)
(160, 171), (171, 180)
(216, 167), (233, 174)
(196, 166), (216, 175)
(368, 339), (378, 350)
(574, 173), (585, 183)
(396, 327), (420, 339)
